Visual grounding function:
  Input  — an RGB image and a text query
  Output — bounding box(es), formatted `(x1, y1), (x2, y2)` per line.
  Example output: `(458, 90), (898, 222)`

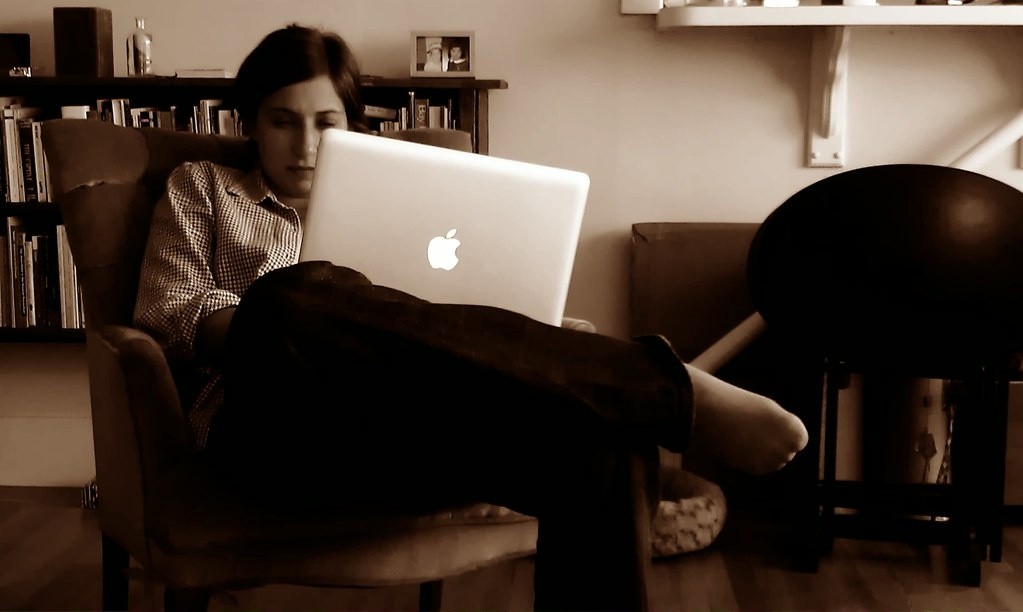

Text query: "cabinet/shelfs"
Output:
(0, 69), (508, 346)
(658, 0), (1022, 170)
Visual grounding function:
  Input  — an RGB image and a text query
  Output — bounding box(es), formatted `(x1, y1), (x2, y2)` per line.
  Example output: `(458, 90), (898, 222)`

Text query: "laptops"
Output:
(298, 130), (590, 331)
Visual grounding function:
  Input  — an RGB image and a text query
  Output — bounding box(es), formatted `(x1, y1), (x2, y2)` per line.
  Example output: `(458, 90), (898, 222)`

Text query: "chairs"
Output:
(738, 162), (1022, 591)
(37, 117), (533, 610)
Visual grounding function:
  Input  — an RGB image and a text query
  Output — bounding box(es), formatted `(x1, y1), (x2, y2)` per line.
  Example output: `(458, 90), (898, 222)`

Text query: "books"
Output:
(0, 89), (460, 326)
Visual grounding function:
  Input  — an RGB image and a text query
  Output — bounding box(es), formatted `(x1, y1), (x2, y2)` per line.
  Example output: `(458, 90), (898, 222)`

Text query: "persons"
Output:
(447, 43), (470, 71)
(423, 42), (443, 72)
(128, 20), (806, 612)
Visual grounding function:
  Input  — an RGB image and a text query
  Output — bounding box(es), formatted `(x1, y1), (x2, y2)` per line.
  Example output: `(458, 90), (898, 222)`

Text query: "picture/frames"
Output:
(408, 29), (477, 81)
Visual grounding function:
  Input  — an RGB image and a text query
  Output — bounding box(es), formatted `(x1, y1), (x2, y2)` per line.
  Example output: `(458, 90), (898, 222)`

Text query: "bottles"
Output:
(126, 17), (152, 78)
